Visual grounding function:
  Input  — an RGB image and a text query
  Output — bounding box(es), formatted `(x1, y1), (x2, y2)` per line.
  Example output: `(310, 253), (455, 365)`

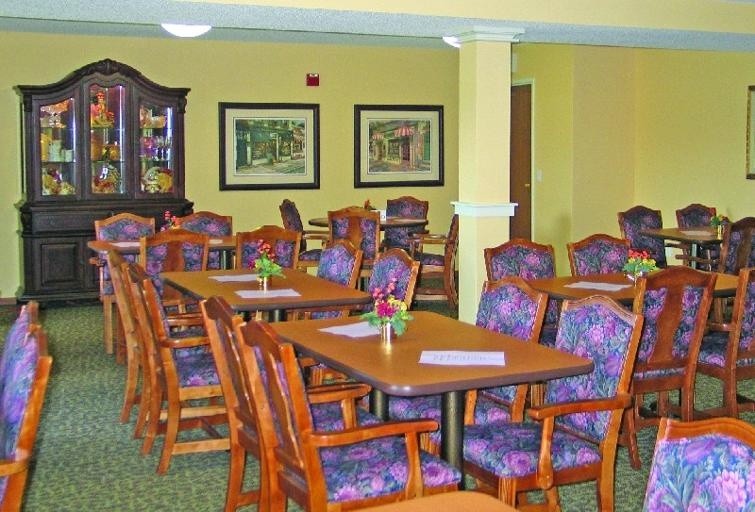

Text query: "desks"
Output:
(342, 489), (519, 510)
(274, 311), (595, 489)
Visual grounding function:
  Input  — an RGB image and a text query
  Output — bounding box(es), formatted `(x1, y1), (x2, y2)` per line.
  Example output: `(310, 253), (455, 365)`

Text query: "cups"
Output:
(60, 150), (72, 162)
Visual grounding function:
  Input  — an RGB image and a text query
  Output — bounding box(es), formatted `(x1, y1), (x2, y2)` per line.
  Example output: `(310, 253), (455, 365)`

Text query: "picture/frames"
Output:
(218, 102), (320, 190)
(354, 104), (444, 187)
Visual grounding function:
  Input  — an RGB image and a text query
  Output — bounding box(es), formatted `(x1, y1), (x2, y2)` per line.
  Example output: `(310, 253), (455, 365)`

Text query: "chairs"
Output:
(1, 299), (40, 392)
(641, 415), (753, 511)
(483, 234), (754, 469)
(237, 319), (462, 508)
(199, 277), (384, 510)
(388, 276), (549, 455)
(1, 324), (52, 512)
(88, 211), (239, 355)
(107, 238), (420, 475)
(463, 294), (643, 510)
(279, 195), (459, 314)
(618, 203), (755, 324)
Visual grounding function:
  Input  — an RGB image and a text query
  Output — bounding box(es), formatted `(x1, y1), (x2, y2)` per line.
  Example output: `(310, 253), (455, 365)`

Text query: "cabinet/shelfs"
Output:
(33, 213), (93, 294)
(32, 95), (82, 193)
(139, 99), (173, 193)
(87, 82), (130, 193)
